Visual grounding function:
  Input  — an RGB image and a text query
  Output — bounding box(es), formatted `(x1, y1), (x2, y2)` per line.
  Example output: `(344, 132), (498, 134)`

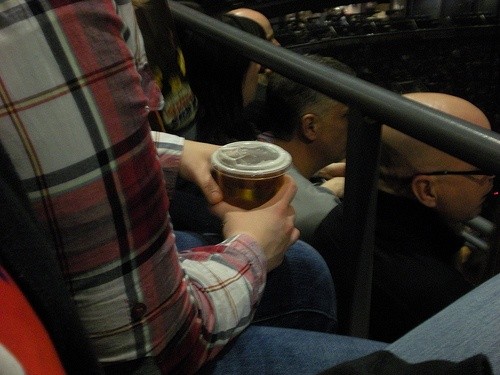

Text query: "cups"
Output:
(213, 140), (294, 211)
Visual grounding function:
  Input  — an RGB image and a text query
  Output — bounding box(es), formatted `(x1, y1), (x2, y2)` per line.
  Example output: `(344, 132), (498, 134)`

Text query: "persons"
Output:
(0, 0), (395, 375)
(252, 53), (356, 242)
(186, 4), (285, 147)
(314, 91), (499, 344)
